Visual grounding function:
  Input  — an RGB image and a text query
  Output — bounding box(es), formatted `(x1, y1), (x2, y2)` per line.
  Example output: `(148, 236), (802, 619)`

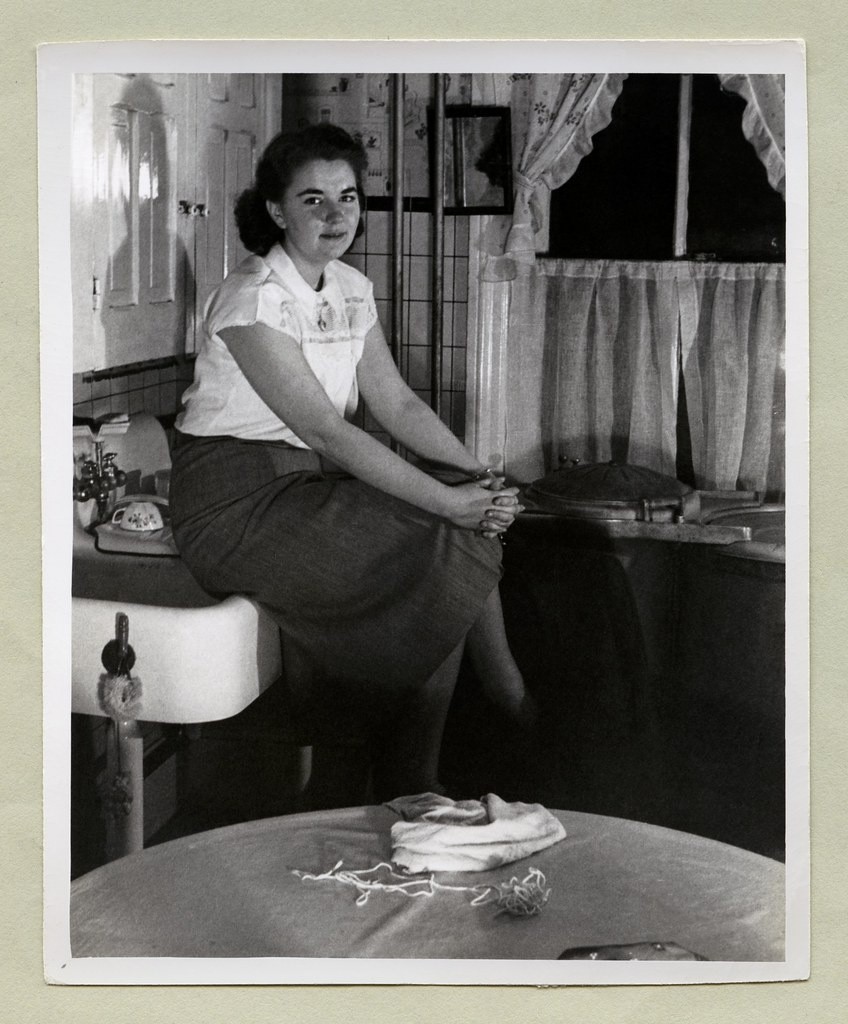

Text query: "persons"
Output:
(167, 124), (552, 805)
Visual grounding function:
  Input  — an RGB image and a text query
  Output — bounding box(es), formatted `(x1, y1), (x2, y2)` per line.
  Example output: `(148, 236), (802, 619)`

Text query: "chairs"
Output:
(523, 519), (788, 849)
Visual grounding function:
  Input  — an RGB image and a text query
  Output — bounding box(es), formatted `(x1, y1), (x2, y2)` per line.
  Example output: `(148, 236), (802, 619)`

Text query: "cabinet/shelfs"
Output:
(71, 72), (284, 373)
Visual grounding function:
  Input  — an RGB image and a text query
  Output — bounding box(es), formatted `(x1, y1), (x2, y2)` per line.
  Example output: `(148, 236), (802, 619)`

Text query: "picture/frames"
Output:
(426, 104), (513, 217)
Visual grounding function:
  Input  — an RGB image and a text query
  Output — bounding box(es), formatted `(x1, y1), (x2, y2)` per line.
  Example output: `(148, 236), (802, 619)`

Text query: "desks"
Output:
(69, 803), (787, 961)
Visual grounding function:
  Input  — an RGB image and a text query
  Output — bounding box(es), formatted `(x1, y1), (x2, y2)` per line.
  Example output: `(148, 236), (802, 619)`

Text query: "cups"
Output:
(111, 499), (164, 532)
(154, 475), (183, 497)
